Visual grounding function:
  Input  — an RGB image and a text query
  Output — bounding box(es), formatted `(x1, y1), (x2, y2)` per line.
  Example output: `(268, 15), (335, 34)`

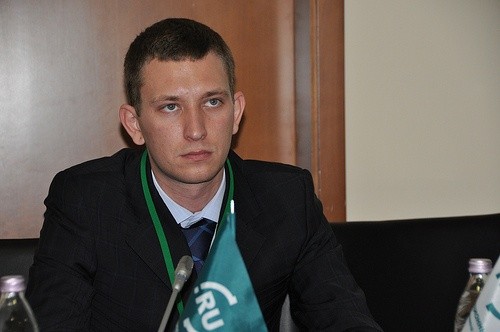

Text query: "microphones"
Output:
(158, 256), (194, 332)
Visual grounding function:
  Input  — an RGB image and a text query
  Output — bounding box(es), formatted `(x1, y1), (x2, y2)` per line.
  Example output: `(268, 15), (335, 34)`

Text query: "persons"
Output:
(13, 17), (384, 332)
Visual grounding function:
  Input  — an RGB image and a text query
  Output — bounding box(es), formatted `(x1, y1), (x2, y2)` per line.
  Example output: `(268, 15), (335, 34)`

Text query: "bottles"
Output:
(0, 275), (38, 332)
(454, 259), (492, 332)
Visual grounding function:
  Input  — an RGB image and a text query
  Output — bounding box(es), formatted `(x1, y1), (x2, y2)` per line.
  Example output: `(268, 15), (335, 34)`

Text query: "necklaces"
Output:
(141, 148), (234, 321)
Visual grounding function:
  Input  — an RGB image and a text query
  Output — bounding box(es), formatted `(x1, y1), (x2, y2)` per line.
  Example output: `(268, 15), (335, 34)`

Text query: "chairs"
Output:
(280, 212), (500, 332)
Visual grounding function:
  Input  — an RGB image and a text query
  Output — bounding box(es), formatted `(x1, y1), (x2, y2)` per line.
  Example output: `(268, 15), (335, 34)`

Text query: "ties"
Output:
(182, 218), (217, 274)
